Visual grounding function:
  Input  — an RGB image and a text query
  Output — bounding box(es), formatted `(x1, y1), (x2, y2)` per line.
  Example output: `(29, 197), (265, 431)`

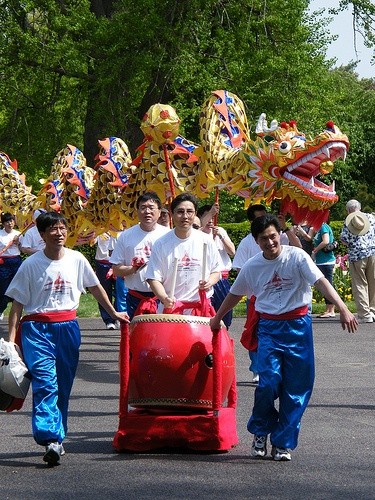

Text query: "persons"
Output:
(5, 212), (130, 466)
(20, 208), (47, 256)
(311, 216), (336, 317)
(90, 230), (121, 330)
(289, 220), (314, 258)
(197, 202), (235, 331)
(109, 191), (171, 323)
(0, 212), (24, 320)
(341, 199), (375, 323)
(108, 231), (129, 312)
(145, 193), (225, 315)
(233, 204), (303, 383)
(157, 208), (170, 228)
(210, 214), (359, 461)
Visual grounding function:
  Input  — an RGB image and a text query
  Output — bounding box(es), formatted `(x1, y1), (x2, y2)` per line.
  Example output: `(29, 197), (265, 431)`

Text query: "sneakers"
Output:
(43, 441), (66, 463)
(354, 314), (373, 323)
(252, 372), (259, 383)
(251, 434), (267, 458)
(107, 322), (115, 330)
(0, 313), (4, 321)
(271, 445), (292, 461)
(114, 320), (120, 330)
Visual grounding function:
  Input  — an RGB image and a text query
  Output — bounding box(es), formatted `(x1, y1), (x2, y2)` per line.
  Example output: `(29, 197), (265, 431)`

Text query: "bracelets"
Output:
(219, 233), (226, 241)
(312, 248), (318, 254)
(282, 227), (290, 234)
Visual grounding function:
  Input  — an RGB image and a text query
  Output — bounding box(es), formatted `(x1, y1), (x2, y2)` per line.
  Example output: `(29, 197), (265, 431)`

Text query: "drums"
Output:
(127, 313), (236, 409)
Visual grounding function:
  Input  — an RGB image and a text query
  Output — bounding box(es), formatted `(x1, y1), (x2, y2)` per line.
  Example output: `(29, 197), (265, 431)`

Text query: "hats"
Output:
(345, 211), (371, 235)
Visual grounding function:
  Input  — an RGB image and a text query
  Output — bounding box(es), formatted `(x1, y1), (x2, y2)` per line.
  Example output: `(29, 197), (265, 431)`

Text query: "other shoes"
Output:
(317, 312), (336, 318)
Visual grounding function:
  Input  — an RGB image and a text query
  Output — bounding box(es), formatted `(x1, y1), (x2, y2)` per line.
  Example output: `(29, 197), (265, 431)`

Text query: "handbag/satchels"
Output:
(322, 241), (339, 254)
(0, 338), (30, 399)
